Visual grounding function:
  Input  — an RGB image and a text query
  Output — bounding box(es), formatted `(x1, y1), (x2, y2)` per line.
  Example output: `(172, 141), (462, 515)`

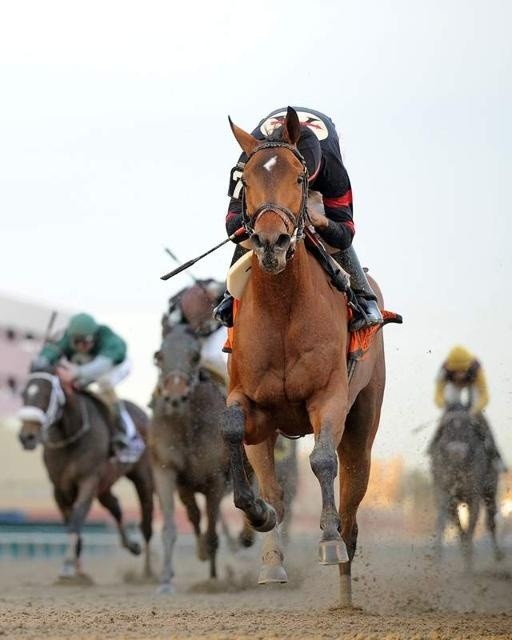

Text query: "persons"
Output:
(215, 106), (383, 334)
(423, 343), (503, 460)
(23, 312), (145, 469)
(151, 281), (232, 405)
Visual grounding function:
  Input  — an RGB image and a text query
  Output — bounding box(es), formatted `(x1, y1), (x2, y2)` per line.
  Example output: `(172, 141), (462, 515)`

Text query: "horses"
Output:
(16, 358), (155, 581)
(425, 399), (504, 562)
(146, 313), (259, 589)
(225, 105), (386, 611)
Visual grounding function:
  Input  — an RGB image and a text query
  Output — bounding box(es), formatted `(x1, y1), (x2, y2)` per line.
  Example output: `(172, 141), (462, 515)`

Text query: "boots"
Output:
(330, 244), (385, 324)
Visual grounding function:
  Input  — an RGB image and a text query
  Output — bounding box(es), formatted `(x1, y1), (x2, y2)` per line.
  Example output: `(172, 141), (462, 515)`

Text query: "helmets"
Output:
(445, 346), (472, 370)
(266, 123), (323, 181)
(66, 312), (98, 336)
